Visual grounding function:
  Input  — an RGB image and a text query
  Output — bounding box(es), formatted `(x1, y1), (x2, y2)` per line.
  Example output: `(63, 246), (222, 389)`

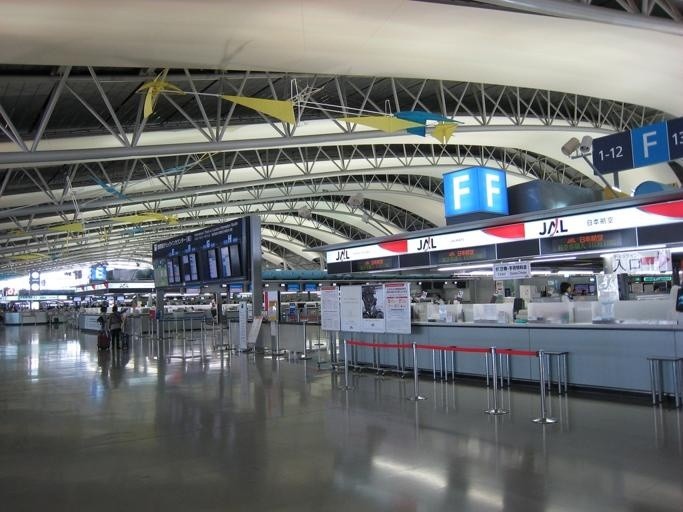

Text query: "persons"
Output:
(512, 296), (527, 321)
(452, 293), (464, 322)
(559, 280), (574, 303)
(98, 306), (110, 339)
(431, 293), (447, 322)
(668, 281), (682, 324)
(408, 288), (424, 321)
(118, 308), (129, 350)
(105, 303), (128, 351)
(96, 329), (111, 352)
(208, 297), (218, 327)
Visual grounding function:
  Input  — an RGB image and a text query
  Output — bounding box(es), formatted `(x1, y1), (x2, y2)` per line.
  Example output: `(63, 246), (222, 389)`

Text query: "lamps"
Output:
(560, 136), (591, 156)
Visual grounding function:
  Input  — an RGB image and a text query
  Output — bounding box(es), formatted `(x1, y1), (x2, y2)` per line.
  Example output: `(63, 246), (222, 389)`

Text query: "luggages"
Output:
(96, 323), (110, 352)
(120, 332), (130, 352)
